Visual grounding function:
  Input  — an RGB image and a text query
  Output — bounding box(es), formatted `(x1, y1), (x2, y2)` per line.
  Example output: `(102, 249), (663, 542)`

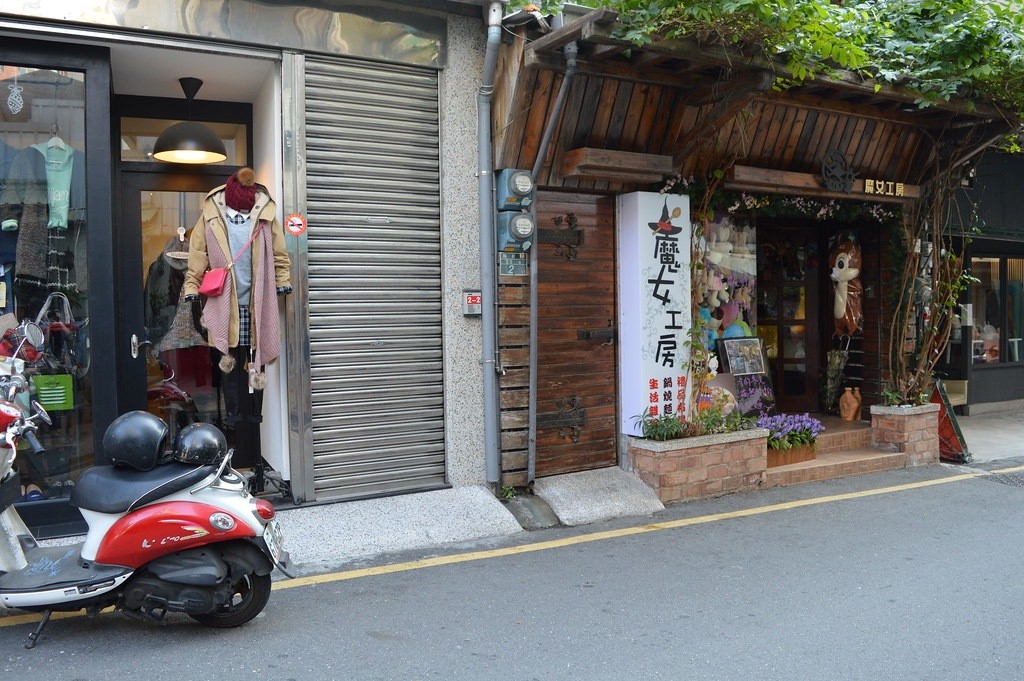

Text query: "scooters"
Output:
(0, 316), (202, 459)
(0, 320), (298, 649)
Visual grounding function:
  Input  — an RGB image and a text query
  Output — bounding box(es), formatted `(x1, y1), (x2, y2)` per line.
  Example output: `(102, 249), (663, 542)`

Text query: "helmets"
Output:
(102, 409), (168, 471)
(173, 423), (228, 464)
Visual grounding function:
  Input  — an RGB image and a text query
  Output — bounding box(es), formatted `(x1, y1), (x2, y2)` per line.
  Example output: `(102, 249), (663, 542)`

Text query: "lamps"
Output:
(152, 76), (227, 164)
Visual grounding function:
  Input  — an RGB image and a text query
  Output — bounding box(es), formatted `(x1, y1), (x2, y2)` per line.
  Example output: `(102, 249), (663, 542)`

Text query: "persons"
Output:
(953, 317), (1013, 363)
(184, 170), (293, 494)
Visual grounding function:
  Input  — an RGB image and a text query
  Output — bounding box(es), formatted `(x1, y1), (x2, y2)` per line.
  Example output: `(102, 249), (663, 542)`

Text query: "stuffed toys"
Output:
(829, 242), (862, 337)
(692, 226), (755, 349)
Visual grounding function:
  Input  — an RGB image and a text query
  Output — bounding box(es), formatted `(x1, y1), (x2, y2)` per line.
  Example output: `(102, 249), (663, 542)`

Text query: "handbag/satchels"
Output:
(15, 292), (90, 379)
(199, 267), (229, 297)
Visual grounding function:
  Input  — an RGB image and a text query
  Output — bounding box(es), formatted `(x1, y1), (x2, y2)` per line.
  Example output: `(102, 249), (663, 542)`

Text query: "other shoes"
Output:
(62, 481), (75, 498)
(26, 484), (42, 501)
(46, 481), (63, 500)
(20, 485), (27, 503)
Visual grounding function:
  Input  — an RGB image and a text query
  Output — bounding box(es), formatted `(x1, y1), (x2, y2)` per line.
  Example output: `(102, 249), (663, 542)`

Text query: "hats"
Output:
(225, 168), (257, 214)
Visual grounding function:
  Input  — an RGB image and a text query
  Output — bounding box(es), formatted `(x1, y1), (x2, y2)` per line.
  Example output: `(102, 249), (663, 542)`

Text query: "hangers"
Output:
(47, 124), (66, 164)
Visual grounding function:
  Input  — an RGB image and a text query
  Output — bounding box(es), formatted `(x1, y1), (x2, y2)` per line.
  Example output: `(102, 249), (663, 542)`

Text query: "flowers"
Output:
(659, 174), (895, 224)
(756, 412), (827, 451)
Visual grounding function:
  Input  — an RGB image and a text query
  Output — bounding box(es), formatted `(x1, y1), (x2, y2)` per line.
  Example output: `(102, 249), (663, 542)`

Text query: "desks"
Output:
(943, 338), (1023, 363)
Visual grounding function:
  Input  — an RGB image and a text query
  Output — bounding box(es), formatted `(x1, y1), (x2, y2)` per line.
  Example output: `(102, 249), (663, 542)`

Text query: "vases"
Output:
(767, 439), (819, 469)
